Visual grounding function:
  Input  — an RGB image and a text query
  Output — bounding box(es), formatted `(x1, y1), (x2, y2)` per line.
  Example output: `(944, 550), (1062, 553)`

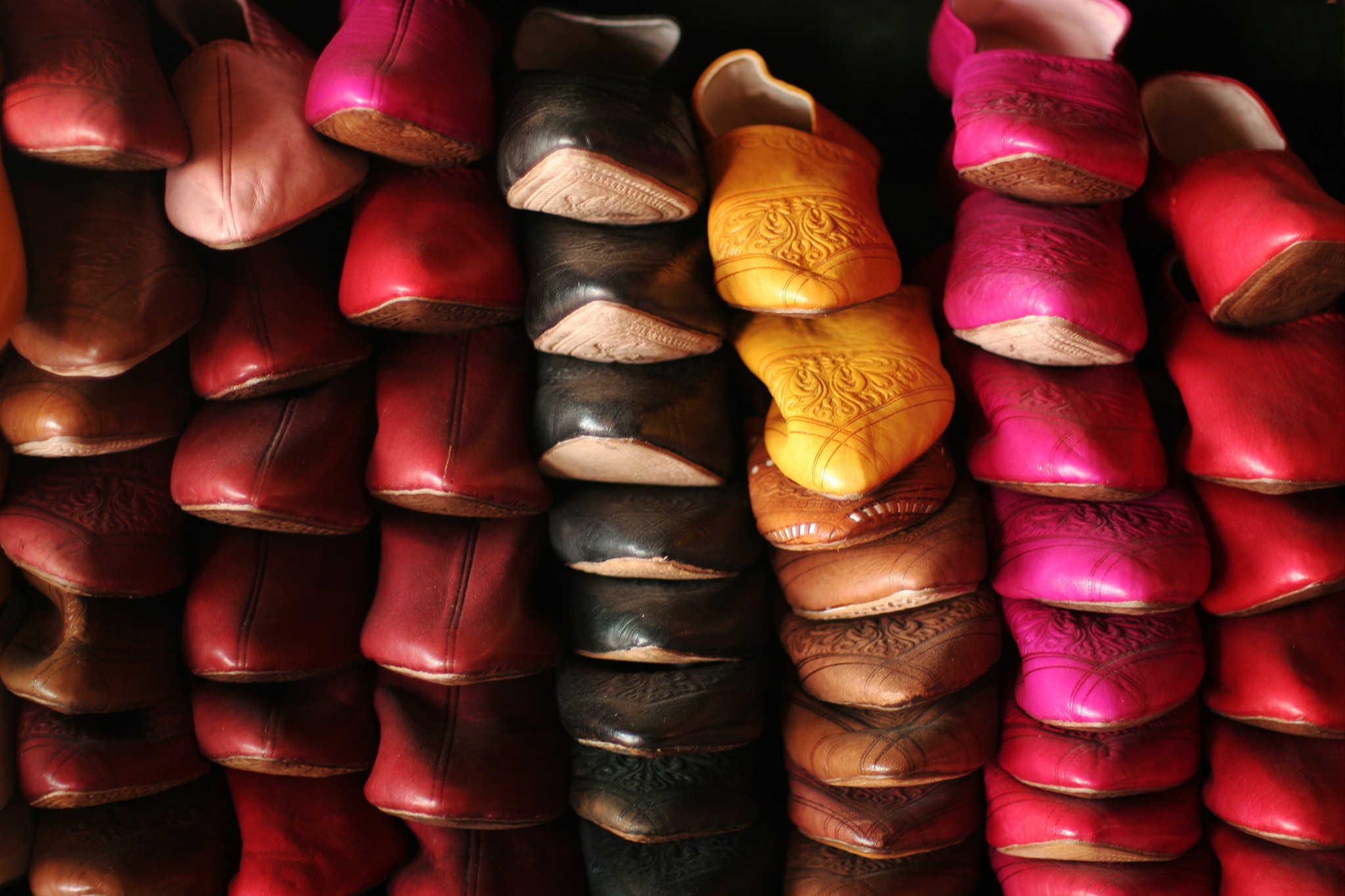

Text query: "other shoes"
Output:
(1186, 470), (1345, 617)
(1205, 622), (1345, 741)
(1150, 251), (1344, 494)
(0, 0), (1220, 896)
(1137, 71), (1344, 327)
(1199, 711), (1345, 851)
(1207, 818), (1345, 895)
